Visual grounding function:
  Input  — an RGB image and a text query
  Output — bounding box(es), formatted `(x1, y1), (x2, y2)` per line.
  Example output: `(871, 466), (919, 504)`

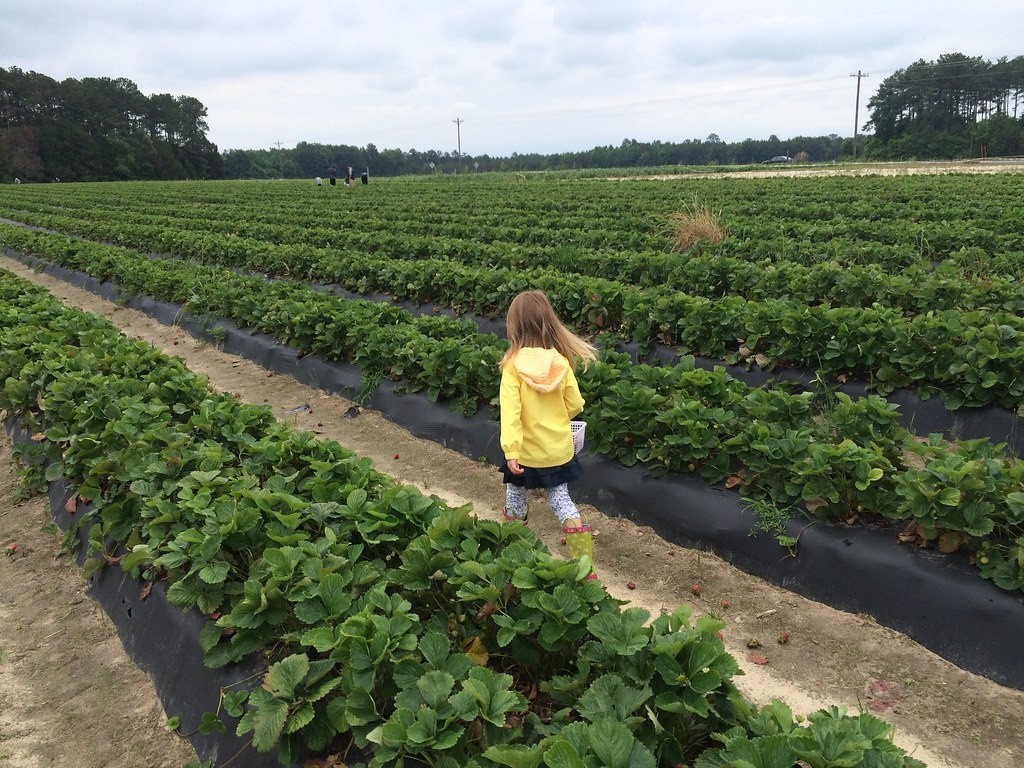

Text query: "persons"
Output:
(498, 291), (597, 582)
(316, 165), (354, 186)
(362, 169), (368, 184)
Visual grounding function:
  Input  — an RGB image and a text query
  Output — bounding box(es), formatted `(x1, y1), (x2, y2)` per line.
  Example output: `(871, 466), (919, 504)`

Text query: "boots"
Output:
(502, 502), (529, 528)
(562, 512), (597, 581)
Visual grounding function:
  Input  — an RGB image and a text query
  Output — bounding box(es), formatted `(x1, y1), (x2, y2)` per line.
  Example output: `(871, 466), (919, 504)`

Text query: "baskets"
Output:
(570, 421), (587, 454)
